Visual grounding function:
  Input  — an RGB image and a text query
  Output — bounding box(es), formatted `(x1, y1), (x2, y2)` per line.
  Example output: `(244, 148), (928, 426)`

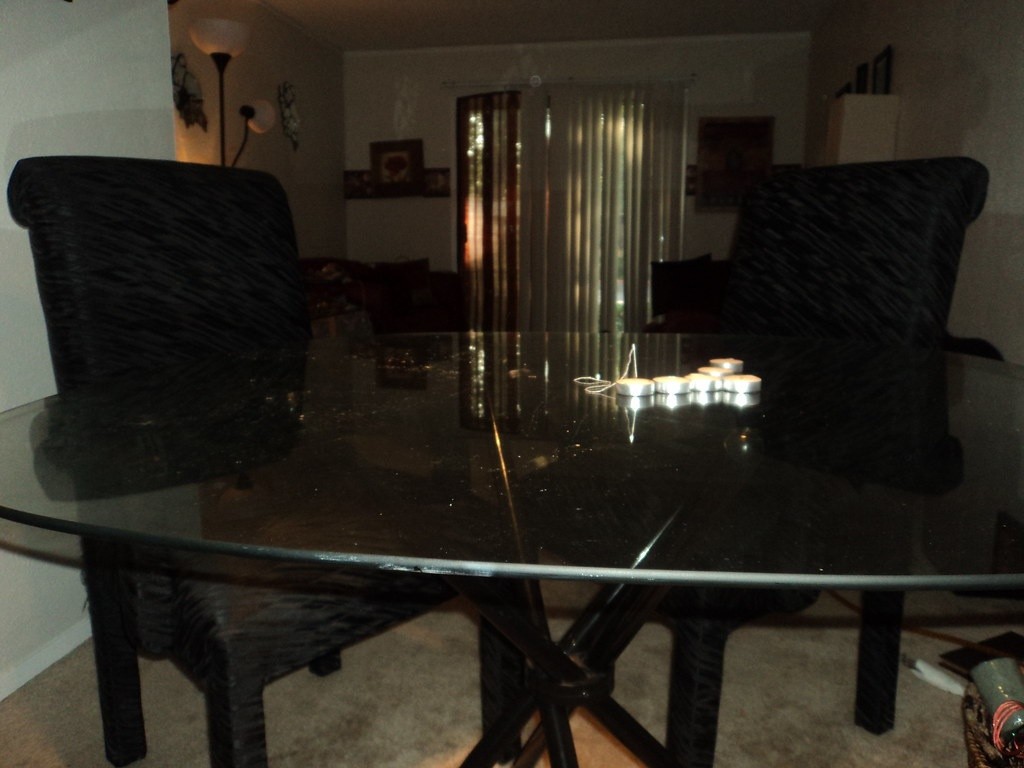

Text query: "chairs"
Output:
(528, 155), (992, 767)
(9, 157), (526, 767)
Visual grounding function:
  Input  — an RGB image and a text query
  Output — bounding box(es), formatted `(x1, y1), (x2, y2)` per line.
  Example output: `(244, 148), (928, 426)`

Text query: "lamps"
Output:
(188, 17), (276, 166)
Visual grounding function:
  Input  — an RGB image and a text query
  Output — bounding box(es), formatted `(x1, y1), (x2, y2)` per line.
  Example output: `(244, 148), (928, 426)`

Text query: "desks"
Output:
(1, 333), (1023, 768)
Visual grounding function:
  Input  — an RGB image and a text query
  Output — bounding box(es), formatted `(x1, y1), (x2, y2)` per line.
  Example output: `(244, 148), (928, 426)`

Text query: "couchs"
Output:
(300, 257), (461, 333)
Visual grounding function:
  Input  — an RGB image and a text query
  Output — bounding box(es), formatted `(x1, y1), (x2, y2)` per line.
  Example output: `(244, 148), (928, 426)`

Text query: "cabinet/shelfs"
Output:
(825, 93), (897, 165)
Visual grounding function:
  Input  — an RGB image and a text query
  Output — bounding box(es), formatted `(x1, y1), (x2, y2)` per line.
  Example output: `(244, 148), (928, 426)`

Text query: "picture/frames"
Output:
(872, 45), (891, 95)
(856, 62), (868, 94)
(369, 139), (422, 198)
